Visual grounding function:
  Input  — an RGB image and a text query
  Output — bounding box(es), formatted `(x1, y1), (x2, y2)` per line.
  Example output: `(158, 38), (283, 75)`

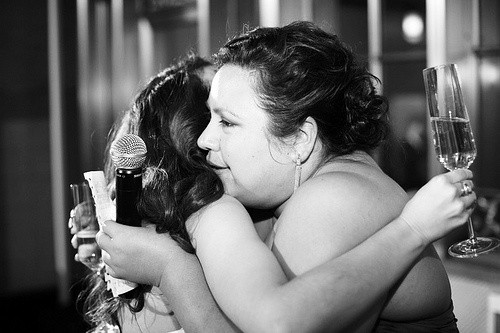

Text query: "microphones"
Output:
(109, 133), (147, 298)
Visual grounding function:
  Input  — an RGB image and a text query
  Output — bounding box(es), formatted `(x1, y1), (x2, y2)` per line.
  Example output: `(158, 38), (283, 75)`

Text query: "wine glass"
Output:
(422, 63), (499, 258)
(69, 183), (120, 333)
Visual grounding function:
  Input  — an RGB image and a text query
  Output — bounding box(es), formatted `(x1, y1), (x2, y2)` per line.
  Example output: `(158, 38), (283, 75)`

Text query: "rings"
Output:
(459, 182), (471, 195)
(67, 210), (78, 228)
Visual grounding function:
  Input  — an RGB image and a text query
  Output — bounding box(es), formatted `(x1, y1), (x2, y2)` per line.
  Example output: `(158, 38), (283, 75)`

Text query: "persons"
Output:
(64, 18), (462, 333)
(80, 56), (477, 333)
